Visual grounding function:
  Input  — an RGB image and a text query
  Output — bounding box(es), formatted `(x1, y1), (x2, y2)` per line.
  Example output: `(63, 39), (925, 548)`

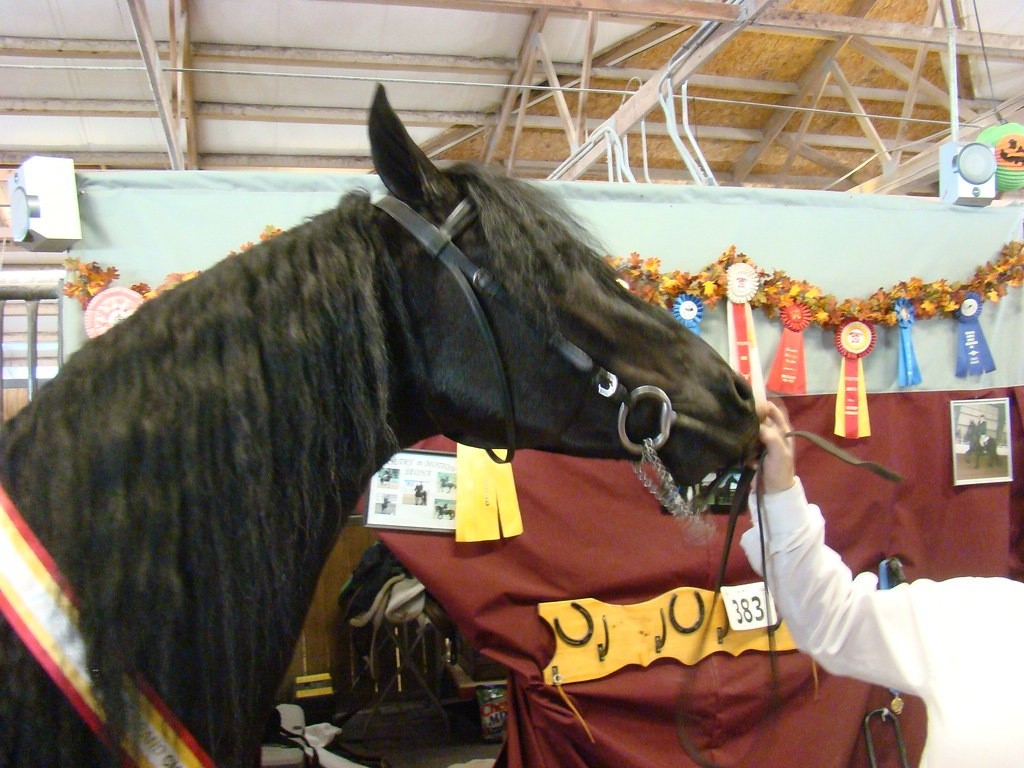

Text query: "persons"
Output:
(738, 401), (1024, 768)
(977, 415), (987, 453)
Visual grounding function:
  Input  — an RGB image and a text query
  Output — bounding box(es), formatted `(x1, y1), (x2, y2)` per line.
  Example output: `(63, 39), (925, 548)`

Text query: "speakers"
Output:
(938, 140), (997, 207)
(9, 156), (82, 252)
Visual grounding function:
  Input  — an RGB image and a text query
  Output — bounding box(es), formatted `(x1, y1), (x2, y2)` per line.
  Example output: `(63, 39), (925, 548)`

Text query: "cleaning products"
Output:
(475, 683), (508, 745)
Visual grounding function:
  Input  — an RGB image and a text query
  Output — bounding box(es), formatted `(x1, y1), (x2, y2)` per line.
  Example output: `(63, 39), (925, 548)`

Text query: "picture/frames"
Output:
(661, 467), (747, 514)
(363, 449), (456, 534)
(950, 397), (1012, 485)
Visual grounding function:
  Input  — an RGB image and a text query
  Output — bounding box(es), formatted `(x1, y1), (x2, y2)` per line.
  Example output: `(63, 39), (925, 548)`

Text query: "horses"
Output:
(0, 75), (764, 768)
